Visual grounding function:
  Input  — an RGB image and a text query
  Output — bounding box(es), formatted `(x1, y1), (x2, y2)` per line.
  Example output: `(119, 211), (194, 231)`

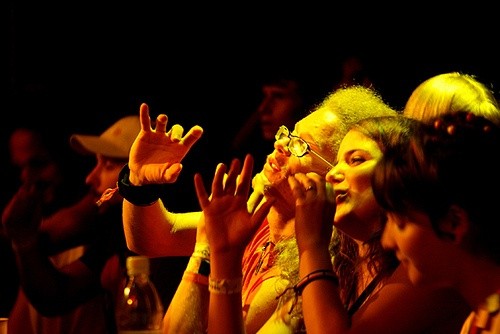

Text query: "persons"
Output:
(371, 110), (500, 334)
(232, 60), (320, 176)
(403, 72), (500, 123)
(2, 116), (142, 334)
(194, 116), (472, 334)
(123, 85), (401, 334)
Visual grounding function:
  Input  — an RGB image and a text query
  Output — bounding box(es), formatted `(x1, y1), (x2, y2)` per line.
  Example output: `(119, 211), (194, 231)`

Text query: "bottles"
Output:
(121, 256), (163, 334)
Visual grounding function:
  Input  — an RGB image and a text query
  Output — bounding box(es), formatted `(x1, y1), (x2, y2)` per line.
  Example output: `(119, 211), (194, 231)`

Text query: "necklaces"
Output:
(344, 261), (389, 319)
(255, 239), (276, 274)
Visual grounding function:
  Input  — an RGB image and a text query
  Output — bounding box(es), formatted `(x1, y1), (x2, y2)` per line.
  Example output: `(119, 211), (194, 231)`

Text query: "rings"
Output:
(304, 186), (316, 193)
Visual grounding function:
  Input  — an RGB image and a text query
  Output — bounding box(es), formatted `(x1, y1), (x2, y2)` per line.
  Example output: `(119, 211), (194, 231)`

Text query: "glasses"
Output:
(275, 125), (335, 169)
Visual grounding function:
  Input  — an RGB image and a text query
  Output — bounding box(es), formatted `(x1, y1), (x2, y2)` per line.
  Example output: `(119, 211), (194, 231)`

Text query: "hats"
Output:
(69, 116), (140, 158)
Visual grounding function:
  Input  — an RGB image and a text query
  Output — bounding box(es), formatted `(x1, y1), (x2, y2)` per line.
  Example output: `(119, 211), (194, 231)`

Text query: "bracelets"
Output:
(181, 244), (210, 286)
(276, 268), (339, 315)
(96, 164), (163, 207)
(208, 275), (242, 295)
